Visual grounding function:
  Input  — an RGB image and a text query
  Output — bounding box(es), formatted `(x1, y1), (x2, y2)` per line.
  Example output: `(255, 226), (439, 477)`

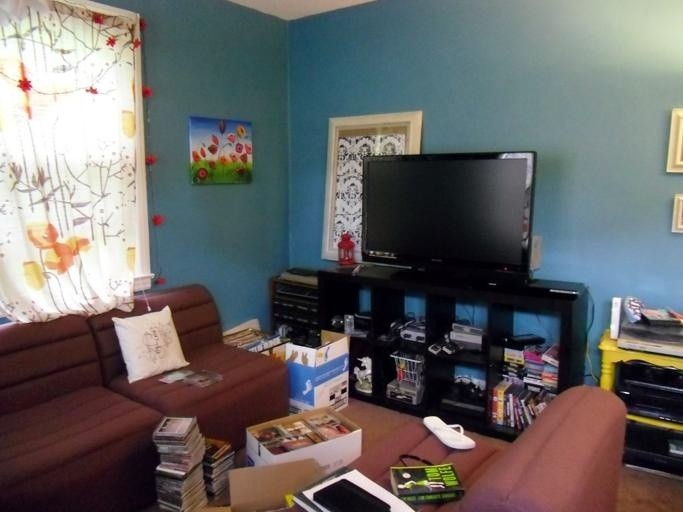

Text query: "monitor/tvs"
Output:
(361, 152), (536, 285)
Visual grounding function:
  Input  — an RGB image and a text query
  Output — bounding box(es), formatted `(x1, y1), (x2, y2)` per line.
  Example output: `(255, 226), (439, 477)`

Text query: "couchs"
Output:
(0, 284), (291, 512)
(268, 385), (627, 512)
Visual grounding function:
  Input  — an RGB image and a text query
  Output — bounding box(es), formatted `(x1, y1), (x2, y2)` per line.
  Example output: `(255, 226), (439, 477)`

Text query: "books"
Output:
(391, 464), (464, 503)
(290, 464), (416, 512)
(153, 416), (236, 512)
(252, 411), (350, 456)
(493, 380), (550, 430)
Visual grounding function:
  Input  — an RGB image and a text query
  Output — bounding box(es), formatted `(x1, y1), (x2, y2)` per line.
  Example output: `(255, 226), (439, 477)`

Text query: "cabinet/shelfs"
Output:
(598, 329), (683, 482)
(317, 263), (589, 442)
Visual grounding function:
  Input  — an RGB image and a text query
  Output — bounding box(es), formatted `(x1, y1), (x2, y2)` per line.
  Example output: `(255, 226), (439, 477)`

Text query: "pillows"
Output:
(111, 305), (191, 384)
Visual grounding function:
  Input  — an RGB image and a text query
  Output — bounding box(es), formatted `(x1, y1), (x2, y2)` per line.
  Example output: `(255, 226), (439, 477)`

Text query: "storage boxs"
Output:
(285, 329), (350, 413)
(193, 458), (328, 512)
(245, 407), (363, 478)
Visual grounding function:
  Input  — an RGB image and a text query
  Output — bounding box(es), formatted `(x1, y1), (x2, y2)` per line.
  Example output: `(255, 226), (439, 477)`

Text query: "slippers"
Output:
(424, 415), (475, 451)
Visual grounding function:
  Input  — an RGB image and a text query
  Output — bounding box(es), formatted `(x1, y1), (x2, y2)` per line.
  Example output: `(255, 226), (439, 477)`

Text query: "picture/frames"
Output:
(665, 108), (683, 173)
(671, 194), (683, 233)
(321, 111), (423, 269)
